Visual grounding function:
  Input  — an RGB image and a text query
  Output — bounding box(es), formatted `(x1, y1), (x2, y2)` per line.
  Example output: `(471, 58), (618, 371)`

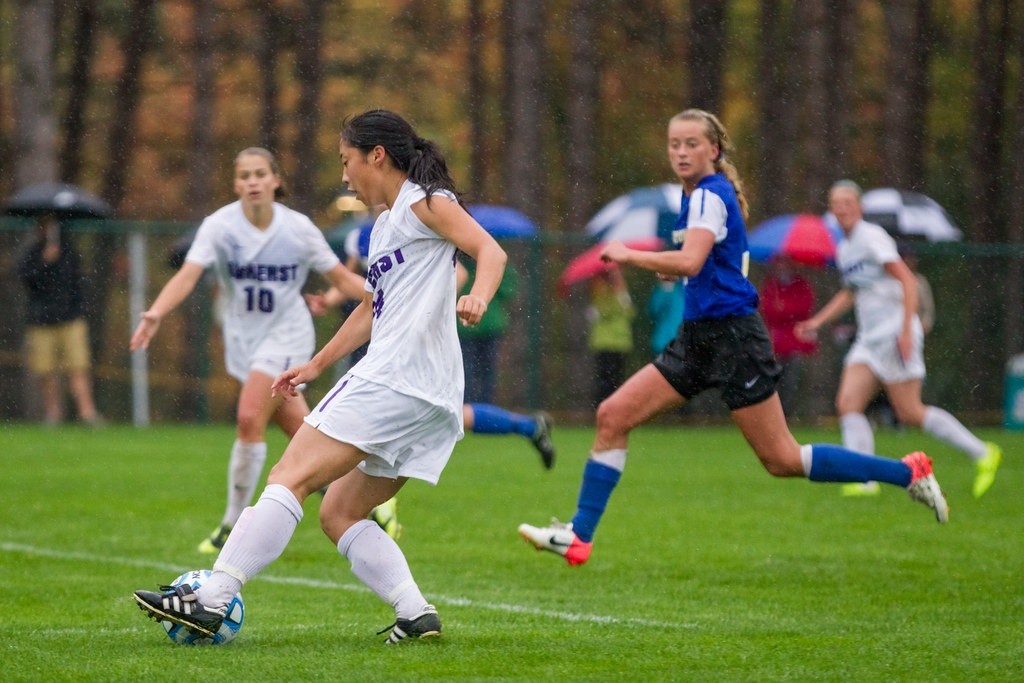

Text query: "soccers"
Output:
(161, 569), (245, 644)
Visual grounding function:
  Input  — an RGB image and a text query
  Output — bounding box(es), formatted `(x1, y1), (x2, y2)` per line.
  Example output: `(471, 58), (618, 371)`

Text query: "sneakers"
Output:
(377, 604), (441, 644)
(133, 584), (223, 639)
(972, 440), (1001, 498)
(532, 410), (557, 469)
(901, 450), (950, 525)
(844, 479), (883, 497)
(371, 498), (401, 542)
(198, 526), (231, 556)
(518, 516), (592, 565)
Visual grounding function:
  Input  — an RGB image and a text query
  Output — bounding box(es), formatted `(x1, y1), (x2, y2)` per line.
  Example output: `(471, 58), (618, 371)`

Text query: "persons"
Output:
(306, 222), (559, 469)
(12, 212), (109, 424)
(645, 277), (687, 356)
(519, 109), (950, 567)
(898, 246), (937, 333)
(756, 263), (817, 413)
(592, 271), (635, 398)
(132, 110), (511, 640)
(454, 248), (520, 403)
(128, 143), (398, 554)
(792, 180), (1003, 498)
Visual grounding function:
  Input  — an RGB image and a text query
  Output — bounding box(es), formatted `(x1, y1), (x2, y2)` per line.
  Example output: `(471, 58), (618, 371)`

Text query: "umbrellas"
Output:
(747, 213), (842, 270)
(0, 180), (115, 219)
(467, 205), (536, 239)
(560, 183), (682, 286)
(860, 187), (964, 243)
